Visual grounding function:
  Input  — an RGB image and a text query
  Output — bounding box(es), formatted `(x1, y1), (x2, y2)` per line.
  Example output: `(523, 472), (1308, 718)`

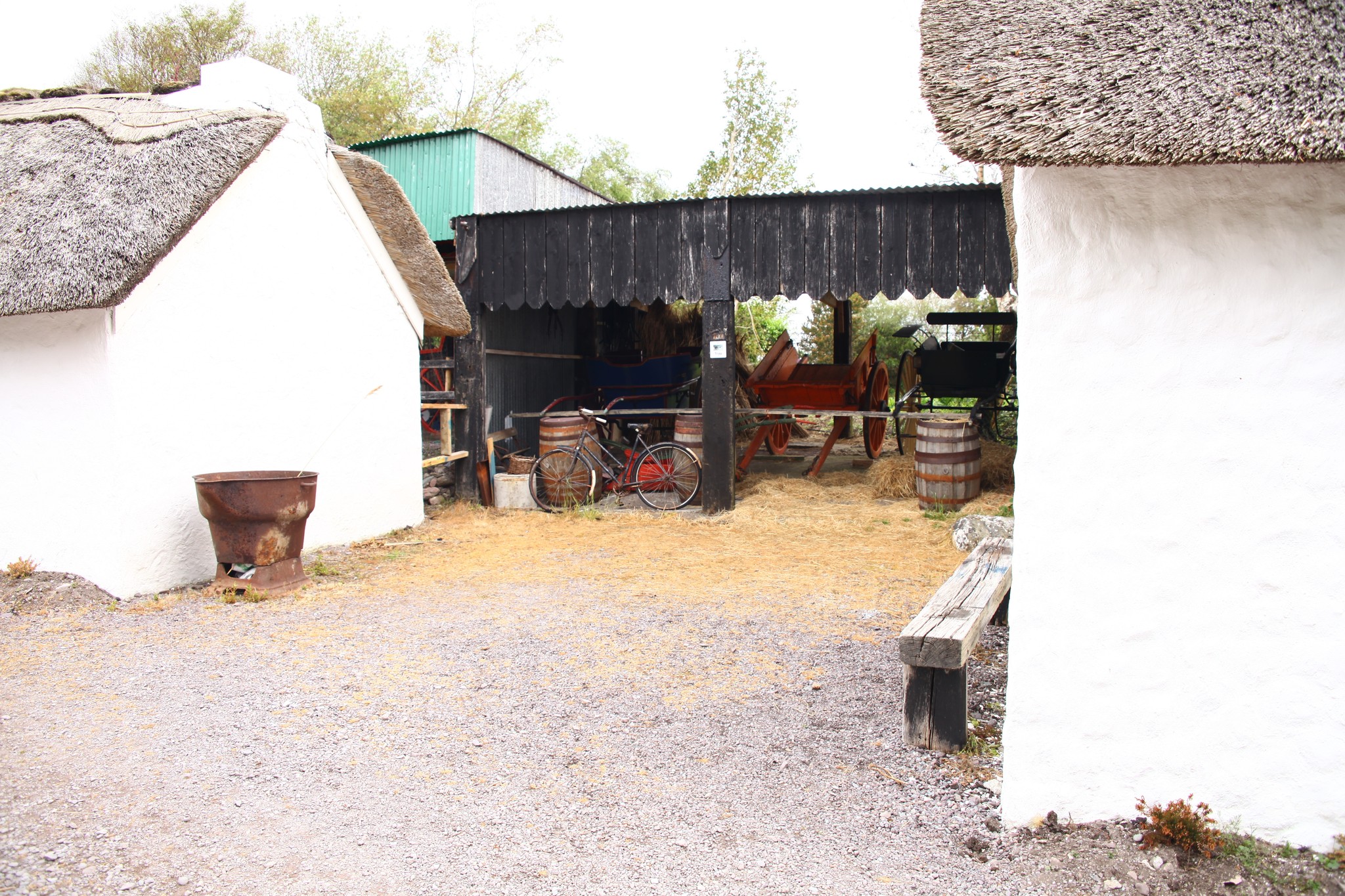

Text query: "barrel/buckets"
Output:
(539, 415), (603, 508)
(914, 418), (982, 515)
(675, 414), (702, 506)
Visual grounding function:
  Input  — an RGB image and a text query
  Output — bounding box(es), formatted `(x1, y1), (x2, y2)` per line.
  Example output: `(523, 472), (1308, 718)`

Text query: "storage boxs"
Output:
(493, 472), (537, 510)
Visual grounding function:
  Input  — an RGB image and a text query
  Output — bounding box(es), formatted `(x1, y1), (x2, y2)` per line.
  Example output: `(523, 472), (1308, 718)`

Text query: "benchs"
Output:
(896, 535), (1013, 753)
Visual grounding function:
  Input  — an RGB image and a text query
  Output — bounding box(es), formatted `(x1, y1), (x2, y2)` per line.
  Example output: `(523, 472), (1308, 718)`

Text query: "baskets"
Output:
(508, 454), (537, 475)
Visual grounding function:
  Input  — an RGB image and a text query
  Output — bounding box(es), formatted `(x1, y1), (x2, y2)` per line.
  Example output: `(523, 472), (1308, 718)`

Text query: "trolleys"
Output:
(733, 328), (892, 482)
(892, 311), (1017, 456)
(539, 353), (704, 462)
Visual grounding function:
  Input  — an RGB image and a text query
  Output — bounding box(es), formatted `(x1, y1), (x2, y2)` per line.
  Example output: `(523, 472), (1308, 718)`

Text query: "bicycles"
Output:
(529, 406), (702, 513)
